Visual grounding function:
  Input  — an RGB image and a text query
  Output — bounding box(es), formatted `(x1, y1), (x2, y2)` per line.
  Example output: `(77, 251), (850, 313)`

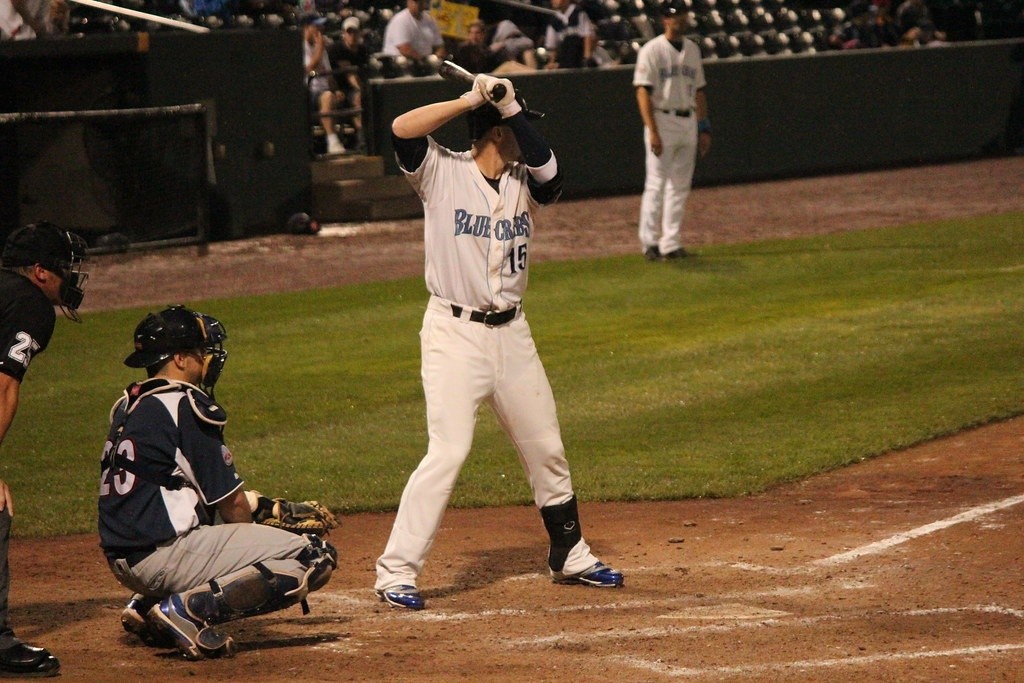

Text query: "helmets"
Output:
(123, 307), (203, 368)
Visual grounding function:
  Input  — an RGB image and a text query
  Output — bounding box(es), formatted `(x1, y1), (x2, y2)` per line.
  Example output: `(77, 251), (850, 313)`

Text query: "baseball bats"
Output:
(438, 60), (507, 99)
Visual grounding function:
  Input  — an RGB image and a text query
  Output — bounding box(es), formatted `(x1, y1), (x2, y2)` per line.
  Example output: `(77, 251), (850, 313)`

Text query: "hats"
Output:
(342, 17), (363, 31)
(1, 225), (71, 270)
(468, 87), (545, 125)
(299, 13), (327, 28)
(661, 1), (693, 15)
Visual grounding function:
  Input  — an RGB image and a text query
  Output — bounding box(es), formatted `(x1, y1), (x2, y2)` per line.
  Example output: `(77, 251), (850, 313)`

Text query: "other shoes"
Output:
(0, 642), (61, 677)
(643, 245), (695, 260)
(327, 135), (346, 154)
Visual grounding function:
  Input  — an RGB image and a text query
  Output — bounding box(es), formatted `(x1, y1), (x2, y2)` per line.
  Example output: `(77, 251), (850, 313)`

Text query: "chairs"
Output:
(108, 0), (938, 133)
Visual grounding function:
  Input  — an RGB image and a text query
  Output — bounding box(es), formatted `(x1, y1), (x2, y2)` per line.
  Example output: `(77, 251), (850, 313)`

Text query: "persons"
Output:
(0, 0), (597, 155)
(831, 0), (947, 49)
(0, 222), (89, 679)
(634, 2), (712, 260)
(98, 305), (339, 660)
(374, 72), (624, 608)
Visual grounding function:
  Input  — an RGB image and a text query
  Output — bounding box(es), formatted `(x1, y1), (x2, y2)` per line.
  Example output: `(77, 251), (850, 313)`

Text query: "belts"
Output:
(450, 305), (517, 328)
(662, 109), (693, 119)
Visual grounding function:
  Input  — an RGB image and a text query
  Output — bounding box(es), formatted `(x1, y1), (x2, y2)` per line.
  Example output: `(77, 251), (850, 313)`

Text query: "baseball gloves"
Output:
(260, 496), (343, 538)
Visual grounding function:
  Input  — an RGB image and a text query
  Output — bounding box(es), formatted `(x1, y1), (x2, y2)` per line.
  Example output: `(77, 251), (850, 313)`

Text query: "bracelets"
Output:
(698, 120), (710, 131)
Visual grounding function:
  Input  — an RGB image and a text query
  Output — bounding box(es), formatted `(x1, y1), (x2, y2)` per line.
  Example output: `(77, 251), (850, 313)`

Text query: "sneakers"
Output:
(378, 585), (428, 611)
(119, 593), (204, 660)
(552, 562), (626, 589)
